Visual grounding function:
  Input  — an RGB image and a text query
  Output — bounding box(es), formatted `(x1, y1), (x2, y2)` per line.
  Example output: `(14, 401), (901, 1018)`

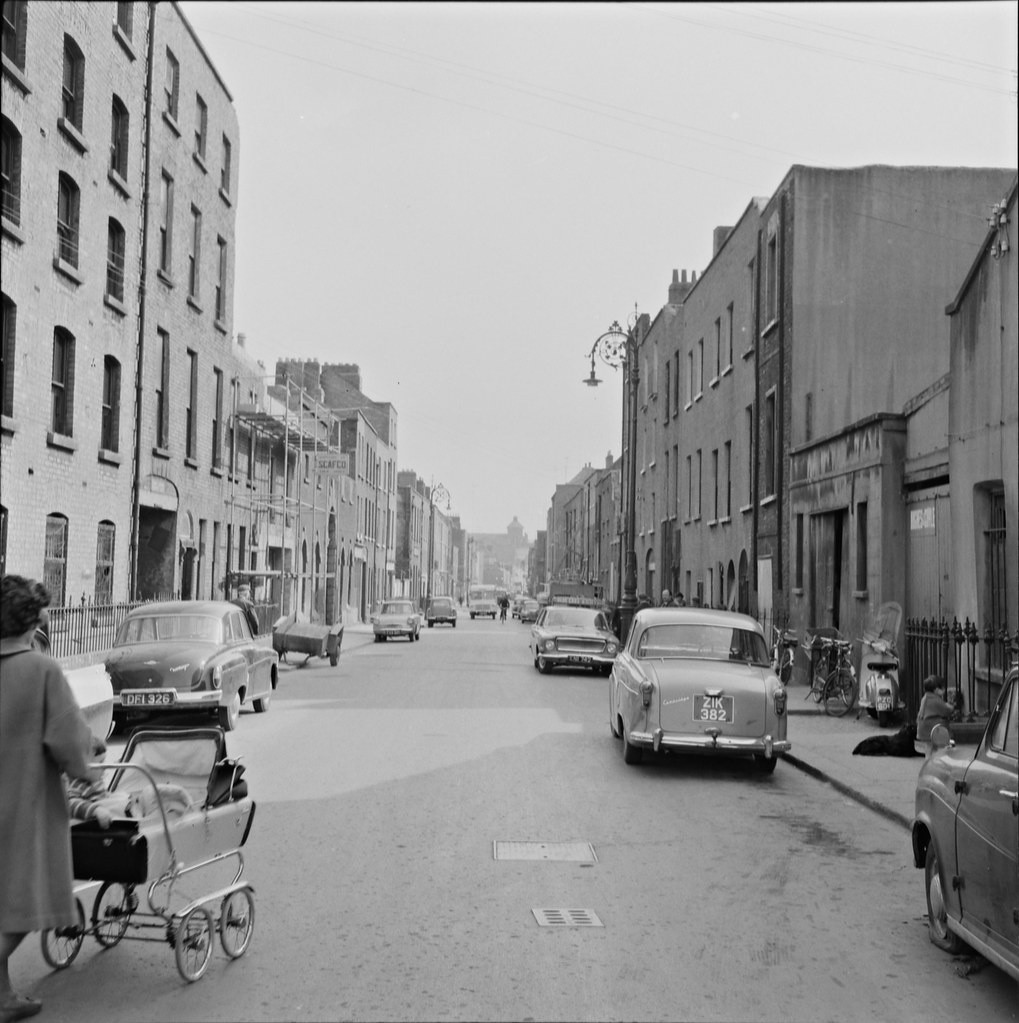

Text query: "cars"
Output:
(372, 597), (424, 643)
(423, 595), (457, 629)
(100, 597), (275, 735)
(511, 595), (543, 624)
(605, 606), (793, 775)
(530, 604), (622, 674)
(909, 661), (1018, 980)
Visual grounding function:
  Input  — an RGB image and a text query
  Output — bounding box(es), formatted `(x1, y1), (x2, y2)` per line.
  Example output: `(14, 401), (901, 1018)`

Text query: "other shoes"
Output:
(0, 996), (43, 1023)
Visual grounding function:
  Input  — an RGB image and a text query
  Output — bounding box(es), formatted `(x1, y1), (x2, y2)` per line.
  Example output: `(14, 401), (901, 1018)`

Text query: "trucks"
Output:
(535, 579), (598, 610)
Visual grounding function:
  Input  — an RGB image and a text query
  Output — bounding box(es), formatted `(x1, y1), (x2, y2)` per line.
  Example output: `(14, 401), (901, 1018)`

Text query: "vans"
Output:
(468, 583), (498, 620)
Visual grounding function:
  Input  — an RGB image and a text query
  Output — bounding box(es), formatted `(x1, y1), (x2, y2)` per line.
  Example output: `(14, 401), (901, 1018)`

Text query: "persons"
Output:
(0, 573), (100, 1021)
(636, 588), (700, 613)
(918, 676), (957, 739)
(65, 732), (114, 829)
(229, 583), (260, 638)
(497, 595), (512, 621)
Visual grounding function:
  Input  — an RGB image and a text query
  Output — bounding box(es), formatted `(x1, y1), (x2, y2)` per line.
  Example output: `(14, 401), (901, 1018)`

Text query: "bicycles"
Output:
(768, 624), (793, 685)
(499, 606), (508, 623)
(803, 630), (862, 716)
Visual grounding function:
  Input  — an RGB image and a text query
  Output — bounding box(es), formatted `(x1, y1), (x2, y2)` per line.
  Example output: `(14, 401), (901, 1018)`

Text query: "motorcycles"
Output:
(856, 635), (906, 726)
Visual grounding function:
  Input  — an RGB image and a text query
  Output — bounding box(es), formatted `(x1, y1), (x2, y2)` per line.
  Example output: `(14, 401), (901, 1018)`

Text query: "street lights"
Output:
(582, 321), (641, 651)
(428, 484), (451, 596)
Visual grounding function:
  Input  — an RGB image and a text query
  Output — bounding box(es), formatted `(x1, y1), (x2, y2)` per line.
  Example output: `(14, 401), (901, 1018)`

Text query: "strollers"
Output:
(40, 723), (262, 988)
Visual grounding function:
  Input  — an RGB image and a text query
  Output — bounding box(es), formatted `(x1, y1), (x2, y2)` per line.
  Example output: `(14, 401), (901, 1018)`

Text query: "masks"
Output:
(30, 623), (50, 652)
